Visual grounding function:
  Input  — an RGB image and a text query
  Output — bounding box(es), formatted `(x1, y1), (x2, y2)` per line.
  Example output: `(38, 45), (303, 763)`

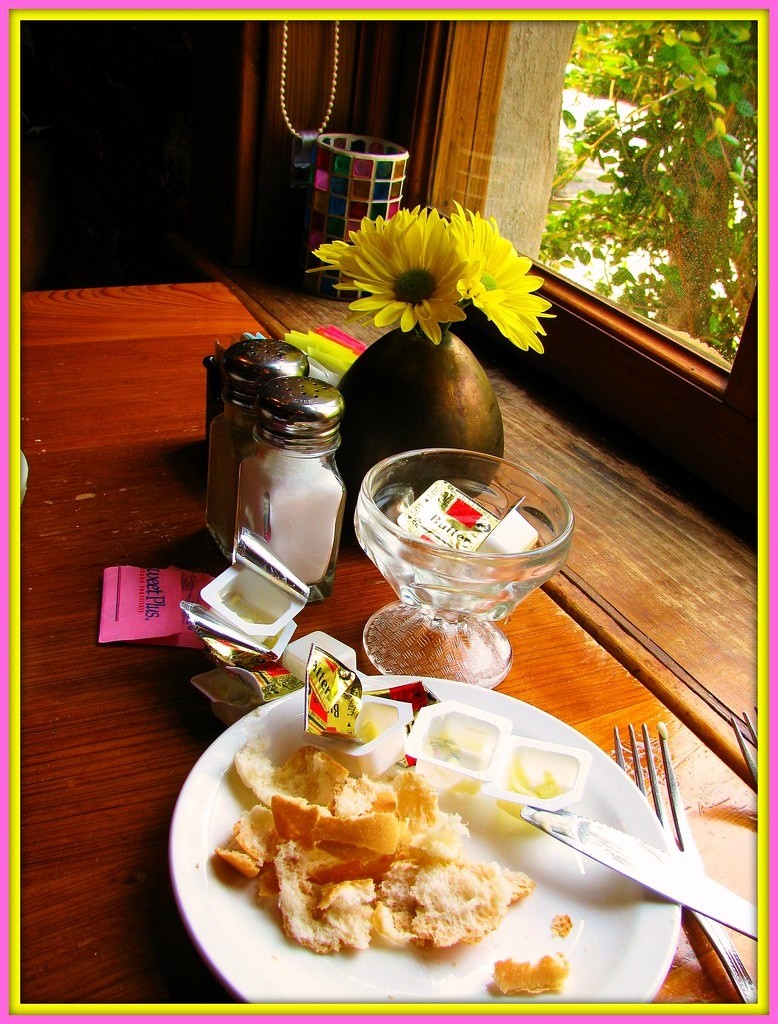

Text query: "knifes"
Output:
(520, 806), (758, 941)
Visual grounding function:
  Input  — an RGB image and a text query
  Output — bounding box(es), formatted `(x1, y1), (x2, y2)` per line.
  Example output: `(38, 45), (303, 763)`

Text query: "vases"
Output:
(332, 326), (505, 519)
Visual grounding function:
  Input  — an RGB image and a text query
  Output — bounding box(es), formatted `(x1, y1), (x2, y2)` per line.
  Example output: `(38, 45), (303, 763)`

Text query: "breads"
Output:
(212, 736), (575, 996)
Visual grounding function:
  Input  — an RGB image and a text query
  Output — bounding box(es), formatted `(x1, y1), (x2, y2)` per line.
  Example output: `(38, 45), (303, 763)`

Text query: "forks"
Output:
(732, 706), (758, 782)
(614, 722), (758, 1005)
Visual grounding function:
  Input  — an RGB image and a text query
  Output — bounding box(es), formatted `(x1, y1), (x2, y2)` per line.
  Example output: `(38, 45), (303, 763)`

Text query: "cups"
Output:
(298, 134), (410, 299)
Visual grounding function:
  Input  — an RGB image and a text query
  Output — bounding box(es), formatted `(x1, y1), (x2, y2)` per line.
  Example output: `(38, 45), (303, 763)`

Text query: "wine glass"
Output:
(354, 449), (575, 690)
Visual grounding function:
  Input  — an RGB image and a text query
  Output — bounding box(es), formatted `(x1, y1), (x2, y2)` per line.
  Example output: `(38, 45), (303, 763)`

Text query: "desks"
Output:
(21, 283), (757, 1003)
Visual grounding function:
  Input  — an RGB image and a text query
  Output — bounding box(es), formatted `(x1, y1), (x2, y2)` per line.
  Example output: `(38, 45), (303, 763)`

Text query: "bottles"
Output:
(208, 339), (348, 603)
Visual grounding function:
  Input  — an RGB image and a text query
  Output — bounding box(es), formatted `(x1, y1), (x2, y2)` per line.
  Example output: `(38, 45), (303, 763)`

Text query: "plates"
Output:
(170, 675), (683, 1004)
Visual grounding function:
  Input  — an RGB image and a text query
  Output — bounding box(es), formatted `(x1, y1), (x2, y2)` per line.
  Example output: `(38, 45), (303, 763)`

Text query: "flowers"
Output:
(303, 197), (558, 356)
